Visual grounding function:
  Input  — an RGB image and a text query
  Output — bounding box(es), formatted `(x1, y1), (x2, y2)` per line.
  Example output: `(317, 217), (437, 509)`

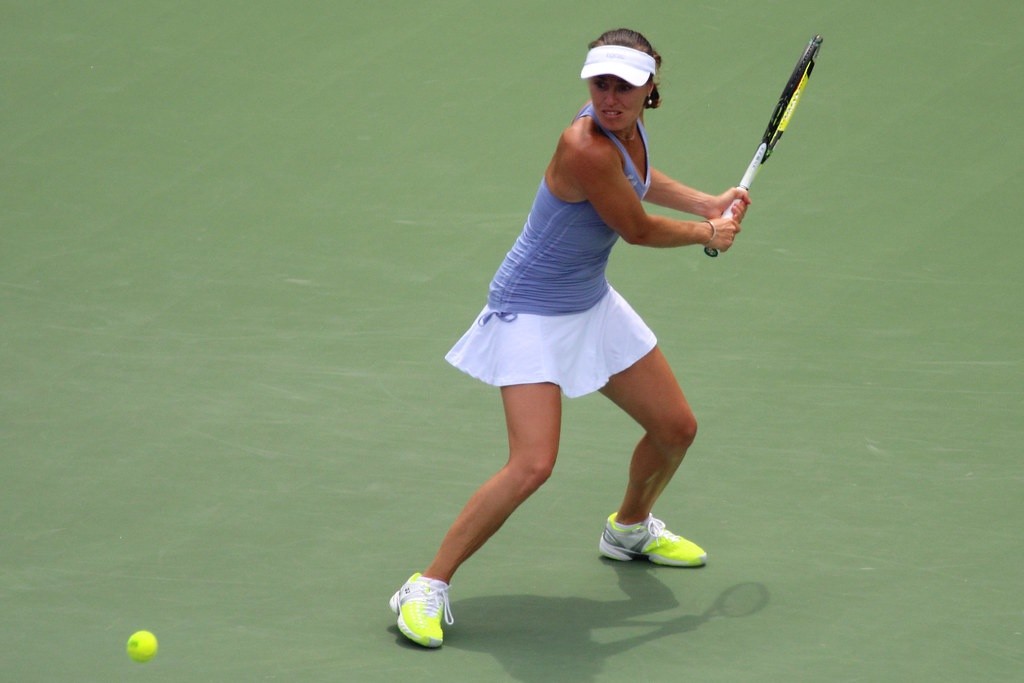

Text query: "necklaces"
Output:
(612, 133), (636, 142)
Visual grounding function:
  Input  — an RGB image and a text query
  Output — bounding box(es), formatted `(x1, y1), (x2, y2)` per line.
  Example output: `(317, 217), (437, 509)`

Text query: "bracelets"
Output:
(700, 220), (716, 247)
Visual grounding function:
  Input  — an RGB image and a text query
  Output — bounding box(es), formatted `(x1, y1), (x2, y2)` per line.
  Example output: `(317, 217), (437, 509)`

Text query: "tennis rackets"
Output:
(703, 34), (825, 258)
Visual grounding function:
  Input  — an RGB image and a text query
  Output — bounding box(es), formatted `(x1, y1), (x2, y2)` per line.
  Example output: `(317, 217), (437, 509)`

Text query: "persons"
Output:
(390, 28), (751, 646)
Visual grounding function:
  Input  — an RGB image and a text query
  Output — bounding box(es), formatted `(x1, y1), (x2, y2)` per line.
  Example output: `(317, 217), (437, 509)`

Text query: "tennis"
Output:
(126, 630), (159, 662)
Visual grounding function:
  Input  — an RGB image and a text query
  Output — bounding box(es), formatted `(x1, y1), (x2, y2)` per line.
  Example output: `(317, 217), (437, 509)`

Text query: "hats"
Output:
(581, 46), (656, 86)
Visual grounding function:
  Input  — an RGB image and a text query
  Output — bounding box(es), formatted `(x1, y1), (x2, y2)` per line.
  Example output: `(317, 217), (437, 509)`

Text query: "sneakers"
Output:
(600, 512), (707, 566)
(389, 573), (454, 648)
